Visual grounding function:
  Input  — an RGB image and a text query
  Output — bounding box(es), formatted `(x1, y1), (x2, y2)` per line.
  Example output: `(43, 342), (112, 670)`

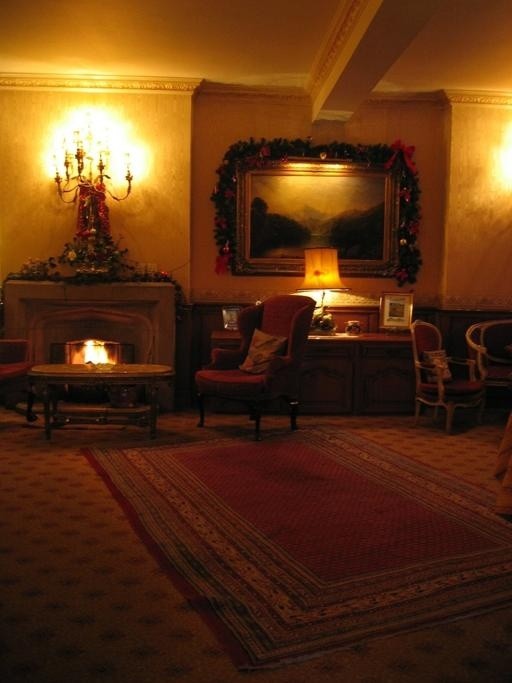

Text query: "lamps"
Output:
(297, 248), (352, 336)
(38, 100), (157, 274)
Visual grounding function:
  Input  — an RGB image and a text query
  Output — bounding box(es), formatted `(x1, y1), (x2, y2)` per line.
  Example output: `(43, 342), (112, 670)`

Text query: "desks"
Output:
(26, 362), (175, 440)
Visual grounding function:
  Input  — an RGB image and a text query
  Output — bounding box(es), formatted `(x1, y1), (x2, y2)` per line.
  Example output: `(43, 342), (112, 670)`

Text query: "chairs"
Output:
(410, 319), (487, 431)
(194, 294), (316, 440)
(466, 320), (512, 426)
(0, 339), (34, 409)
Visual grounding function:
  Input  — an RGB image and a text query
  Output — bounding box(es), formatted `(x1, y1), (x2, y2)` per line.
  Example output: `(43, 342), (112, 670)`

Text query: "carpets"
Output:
(79, 422), (512, 672)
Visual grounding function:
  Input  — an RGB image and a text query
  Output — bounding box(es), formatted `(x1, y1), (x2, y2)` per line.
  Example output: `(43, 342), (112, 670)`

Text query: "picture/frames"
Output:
(234, 156), (402, 276)
(378, 290), (415, 331)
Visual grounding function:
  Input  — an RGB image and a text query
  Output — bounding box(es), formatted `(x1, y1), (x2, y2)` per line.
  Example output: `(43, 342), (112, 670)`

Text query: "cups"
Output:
(345, 320), (360, 336)
